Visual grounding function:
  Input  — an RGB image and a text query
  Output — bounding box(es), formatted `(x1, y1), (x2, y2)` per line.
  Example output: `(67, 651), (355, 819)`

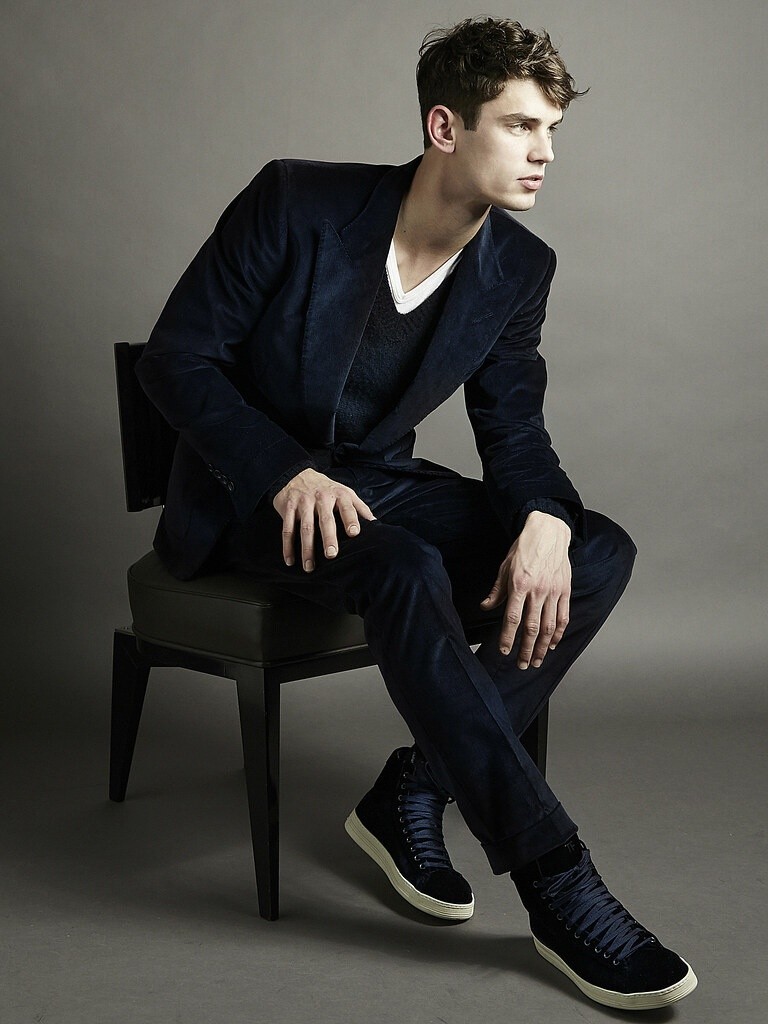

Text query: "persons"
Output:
(133, 18), (698, 1011)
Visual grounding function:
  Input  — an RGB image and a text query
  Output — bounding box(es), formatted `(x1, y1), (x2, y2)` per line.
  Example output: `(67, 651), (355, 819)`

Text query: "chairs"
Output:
(100, 342), (550, 922)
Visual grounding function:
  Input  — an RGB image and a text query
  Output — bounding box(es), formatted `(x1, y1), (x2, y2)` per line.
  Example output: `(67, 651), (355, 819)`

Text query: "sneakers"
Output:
(510, 832), (699, 1009)
(345, 746), (475, 920)
